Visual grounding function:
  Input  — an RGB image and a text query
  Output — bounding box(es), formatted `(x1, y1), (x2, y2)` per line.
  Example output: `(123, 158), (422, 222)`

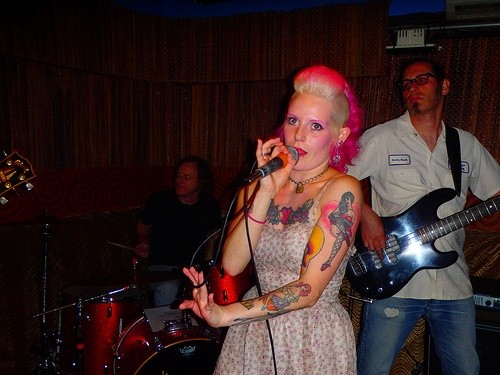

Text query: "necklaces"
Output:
(287, 165), (331, 194)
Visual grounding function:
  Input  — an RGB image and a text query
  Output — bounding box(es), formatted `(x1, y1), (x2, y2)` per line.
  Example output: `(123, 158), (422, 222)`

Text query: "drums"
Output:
(59, 293), (143, 375)
(186, 258), (255, 306)
(110, 307), (228, 375)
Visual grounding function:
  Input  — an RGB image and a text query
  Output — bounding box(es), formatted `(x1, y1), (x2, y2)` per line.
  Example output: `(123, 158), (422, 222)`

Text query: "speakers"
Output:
(427, 327), (500, 375)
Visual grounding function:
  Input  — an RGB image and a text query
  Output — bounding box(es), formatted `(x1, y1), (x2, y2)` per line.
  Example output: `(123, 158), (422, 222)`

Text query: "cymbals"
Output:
(75, 265), (185, 285)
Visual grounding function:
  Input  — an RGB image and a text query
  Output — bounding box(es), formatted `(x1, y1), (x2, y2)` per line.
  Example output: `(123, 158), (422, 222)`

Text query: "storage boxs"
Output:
(423, 307), (500, 375)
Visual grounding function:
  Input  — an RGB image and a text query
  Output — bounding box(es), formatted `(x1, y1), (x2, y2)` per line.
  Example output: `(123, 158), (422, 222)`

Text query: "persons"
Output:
(347, 57), (500, 375)
(113, 156), (217, 325)
(179, 66), (362, 375)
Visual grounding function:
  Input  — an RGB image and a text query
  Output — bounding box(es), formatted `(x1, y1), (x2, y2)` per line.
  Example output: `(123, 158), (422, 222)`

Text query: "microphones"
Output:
(243, 146), (298, 185)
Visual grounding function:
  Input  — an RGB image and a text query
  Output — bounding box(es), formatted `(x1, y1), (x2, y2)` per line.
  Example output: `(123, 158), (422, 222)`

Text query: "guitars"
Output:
(0, 149), (37, 206)
(345, 187), (500, 300)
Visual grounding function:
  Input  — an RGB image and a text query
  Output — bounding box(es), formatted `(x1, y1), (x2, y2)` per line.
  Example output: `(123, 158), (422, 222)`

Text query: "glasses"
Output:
(177, 173), (198, 180)
(400, 72), (441, 90)
